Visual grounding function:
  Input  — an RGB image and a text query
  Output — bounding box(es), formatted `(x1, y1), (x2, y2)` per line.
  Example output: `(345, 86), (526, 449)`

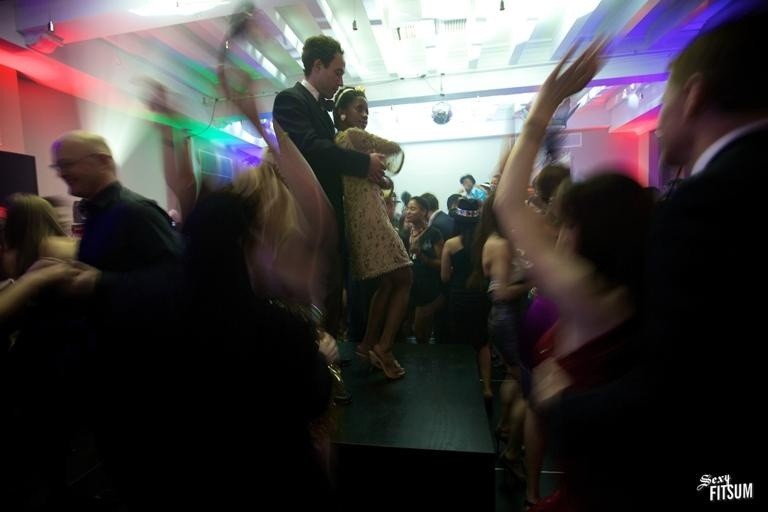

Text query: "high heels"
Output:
(495, 424), (526, 485)
(356, 349), (407, 379)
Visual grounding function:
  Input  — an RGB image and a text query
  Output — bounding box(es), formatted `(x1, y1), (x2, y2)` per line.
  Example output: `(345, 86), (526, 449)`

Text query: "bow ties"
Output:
(318, 93), (334, 111)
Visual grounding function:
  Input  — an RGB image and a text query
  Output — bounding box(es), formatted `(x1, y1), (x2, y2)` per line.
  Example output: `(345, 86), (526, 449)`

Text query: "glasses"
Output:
(48, 152), (94, 172)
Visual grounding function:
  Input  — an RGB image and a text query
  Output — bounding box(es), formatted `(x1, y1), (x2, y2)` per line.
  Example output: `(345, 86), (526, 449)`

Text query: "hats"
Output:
(449, 200), (480, 222)
(460, 175), (475, 184)
(392, 192), (401, 203)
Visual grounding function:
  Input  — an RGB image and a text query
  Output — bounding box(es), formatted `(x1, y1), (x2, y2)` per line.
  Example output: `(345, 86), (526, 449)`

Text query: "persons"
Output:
(1, 0), (767, 512)
